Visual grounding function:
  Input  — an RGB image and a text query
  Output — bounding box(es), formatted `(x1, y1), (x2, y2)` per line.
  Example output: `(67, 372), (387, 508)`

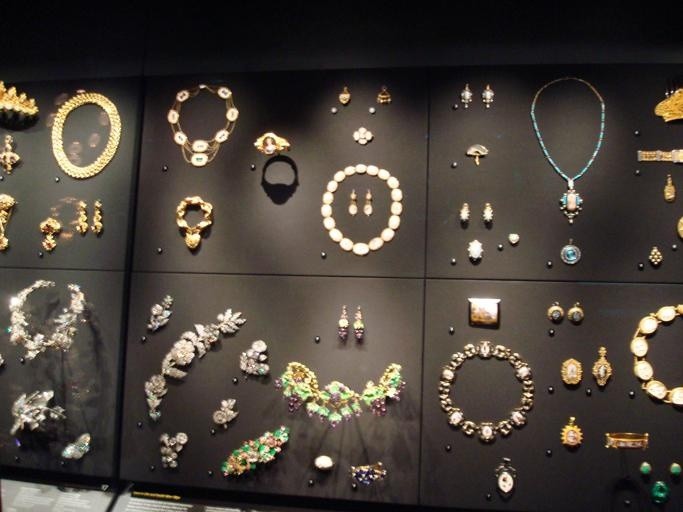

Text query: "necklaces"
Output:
(321, 164), (402, 255)
(167, 85), (238, 166)
(531, 77), (606, 222)
(631, 303), (682, 407)
(52, 94), (122, 178)
(438, 339), (533, 441)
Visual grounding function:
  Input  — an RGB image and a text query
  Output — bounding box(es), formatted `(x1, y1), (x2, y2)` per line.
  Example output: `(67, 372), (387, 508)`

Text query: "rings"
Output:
(253, 132), (292, 156)
(175, 196), (214, 250)
(350, 466), (389, 487)
(607, 431), (651, 451)
